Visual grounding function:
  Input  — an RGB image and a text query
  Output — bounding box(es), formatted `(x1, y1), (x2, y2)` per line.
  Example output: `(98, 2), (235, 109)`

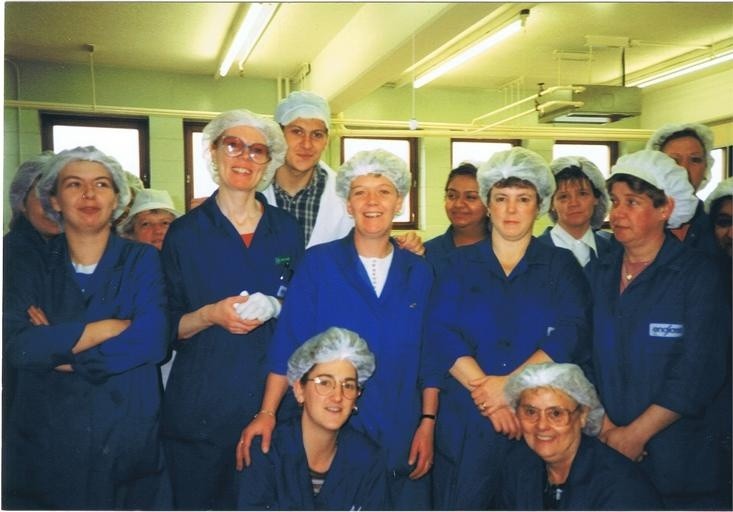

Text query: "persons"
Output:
(5, 90), (732, 510)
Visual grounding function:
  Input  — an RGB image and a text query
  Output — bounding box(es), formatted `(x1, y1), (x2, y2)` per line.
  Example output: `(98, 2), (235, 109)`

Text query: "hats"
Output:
(606, 148), (698, 229)
(275, 89), (332, 133)
(116, 188), (186, 237)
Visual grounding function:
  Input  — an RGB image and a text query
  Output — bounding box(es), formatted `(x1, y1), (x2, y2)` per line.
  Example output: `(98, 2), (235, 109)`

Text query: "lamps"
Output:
(213, 4), (278, 81)
(416, 8), (531, 93)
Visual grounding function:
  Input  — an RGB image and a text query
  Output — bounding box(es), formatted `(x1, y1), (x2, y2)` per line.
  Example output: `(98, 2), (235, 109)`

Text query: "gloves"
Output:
(236, 292), (281, 323)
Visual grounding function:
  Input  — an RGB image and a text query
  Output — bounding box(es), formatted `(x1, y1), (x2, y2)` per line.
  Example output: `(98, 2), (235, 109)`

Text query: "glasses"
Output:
(523, 403), (580, 426)
(306, 374), (363, 400)
(220, 136), (273, 165)
